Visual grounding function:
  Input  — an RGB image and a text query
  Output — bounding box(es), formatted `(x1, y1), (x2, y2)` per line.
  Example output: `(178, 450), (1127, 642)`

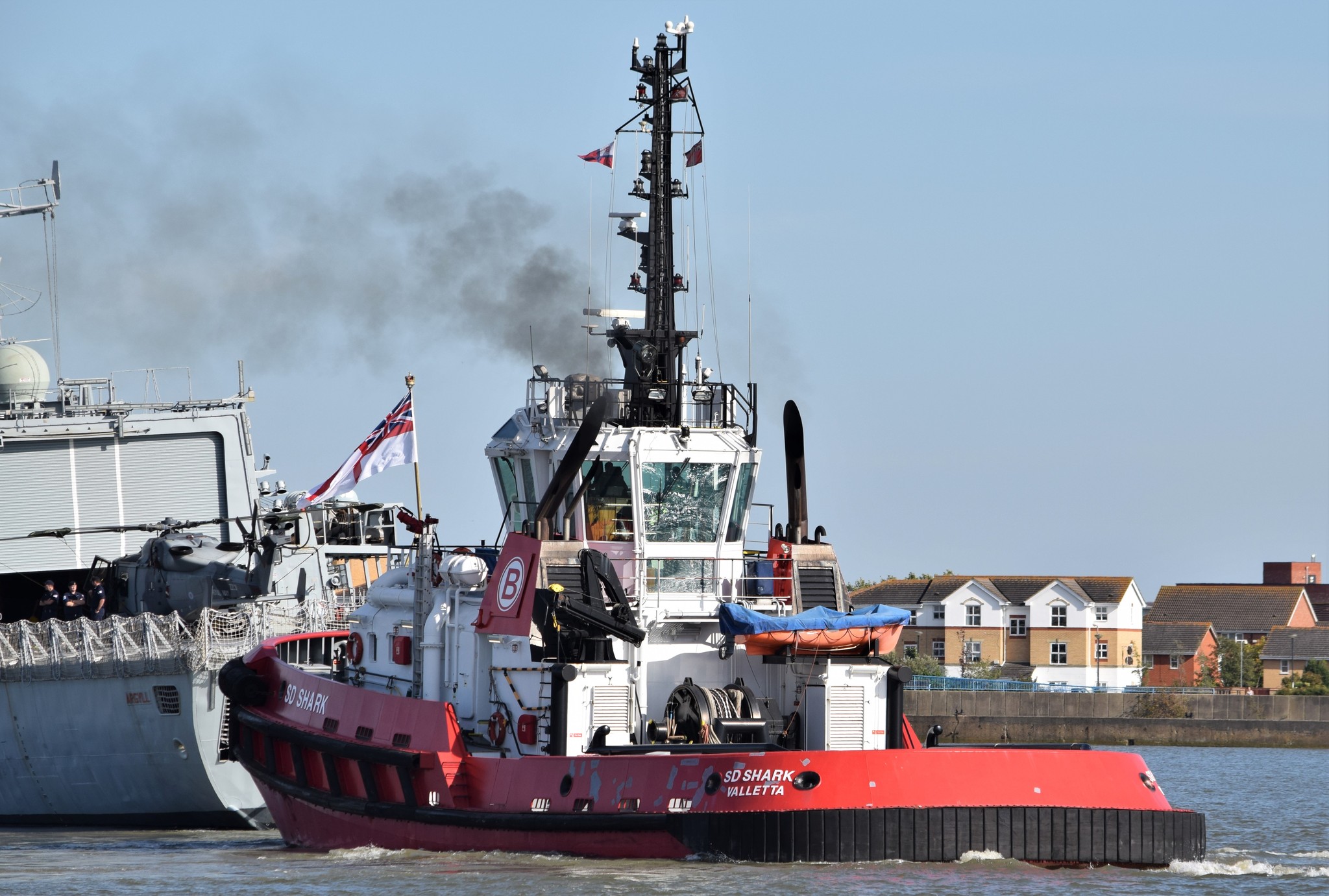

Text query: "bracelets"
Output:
(71, 599), (73, 602)
(96, 608), (100, 611)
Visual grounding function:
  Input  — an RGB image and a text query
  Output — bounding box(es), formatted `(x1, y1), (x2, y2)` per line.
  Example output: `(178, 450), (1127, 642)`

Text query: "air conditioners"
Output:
(602, 389), (632, 419)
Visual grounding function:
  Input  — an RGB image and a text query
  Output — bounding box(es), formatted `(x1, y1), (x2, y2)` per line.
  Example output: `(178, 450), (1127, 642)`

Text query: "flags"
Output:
(295, 388), (418, 511)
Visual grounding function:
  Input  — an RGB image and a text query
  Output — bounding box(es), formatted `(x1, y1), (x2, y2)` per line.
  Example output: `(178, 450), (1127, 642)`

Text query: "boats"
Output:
(0, 163), (428, 830)
(716, 602), (910, 657)
(216, 20), (1208, 872)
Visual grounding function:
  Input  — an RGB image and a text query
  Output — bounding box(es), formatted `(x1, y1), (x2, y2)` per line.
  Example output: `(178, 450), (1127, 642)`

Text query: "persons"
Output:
(39, 580), (59, 622)
(662, 466), (689, 541)
(592, 461), (629, 499)
(88, 576), (107, 621)
(63, 581), (85, 621)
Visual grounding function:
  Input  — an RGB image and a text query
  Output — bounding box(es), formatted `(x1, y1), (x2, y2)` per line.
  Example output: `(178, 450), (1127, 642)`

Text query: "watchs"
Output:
(43, 600), (44, 603)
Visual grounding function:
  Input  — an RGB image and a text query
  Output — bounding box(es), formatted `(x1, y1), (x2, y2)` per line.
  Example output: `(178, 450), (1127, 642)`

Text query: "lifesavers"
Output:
(487, 711), (506, 747)
(336, 607), (345, 621)
(346, 632), (363, 666)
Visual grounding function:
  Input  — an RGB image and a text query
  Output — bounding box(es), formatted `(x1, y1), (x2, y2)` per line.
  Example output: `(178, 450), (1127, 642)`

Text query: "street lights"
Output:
(914, 631), (923, 654)
(1240, 639), (1248, 688)
(1289, 634), (1297, 688)
(1094, 634), (1103, 687)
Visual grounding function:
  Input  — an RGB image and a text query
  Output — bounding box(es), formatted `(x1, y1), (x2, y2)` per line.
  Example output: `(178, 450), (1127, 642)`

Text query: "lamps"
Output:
(533, 364), (548, 379)
(691, 368), (714, 403)
(632, 340), (658, 382)
(531, 419), (553, 444)
(258, 481), (292, 565)
(648, 389), (667, 400)
